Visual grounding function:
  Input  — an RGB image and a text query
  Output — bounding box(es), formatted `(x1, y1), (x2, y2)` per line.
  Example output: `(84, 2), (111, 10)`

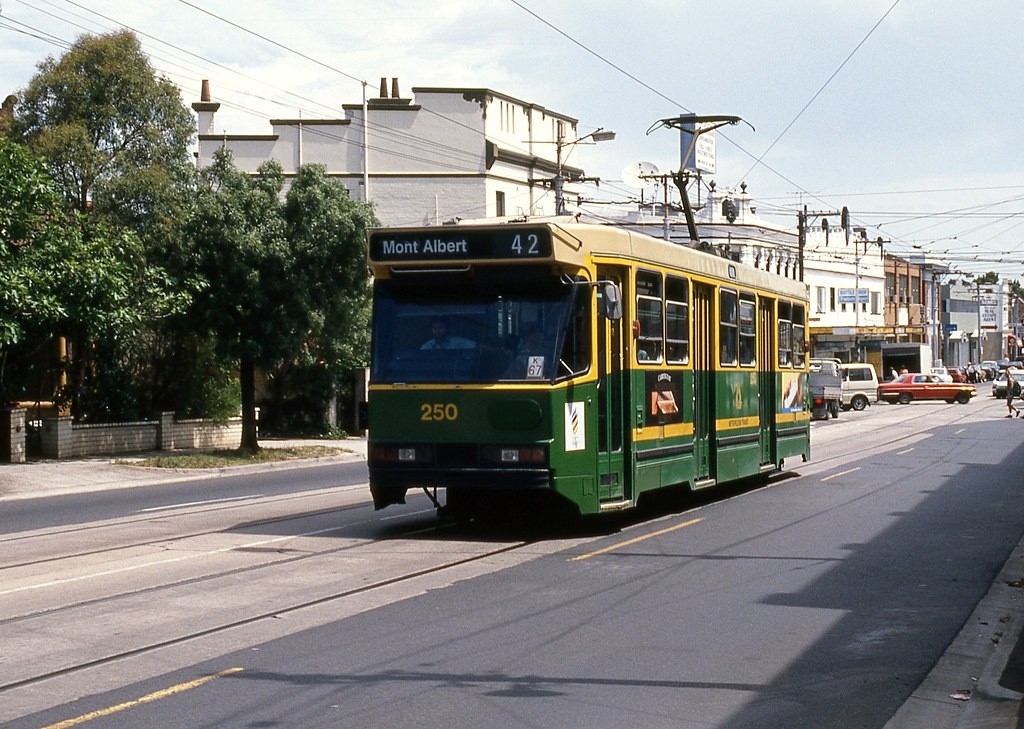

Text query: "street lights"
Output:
(555, 126), (616, 215)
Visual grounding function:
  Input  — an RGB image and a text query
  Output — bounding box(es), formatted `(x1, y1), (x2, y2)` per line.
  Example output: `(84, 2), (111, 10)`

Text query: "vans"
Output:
(837, 363), (881, 411)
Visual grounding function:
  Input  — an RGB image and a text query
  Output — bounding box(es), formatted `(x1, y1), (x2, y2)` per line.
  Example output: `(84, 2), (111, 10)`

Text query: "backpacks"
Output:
(1008, 378), (1021, 396)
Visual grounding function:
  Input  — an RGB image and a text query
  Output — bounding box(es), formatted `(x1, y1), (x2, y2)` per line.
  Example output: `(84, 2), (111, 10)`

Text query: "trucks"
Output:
(808, 357), (843, 419)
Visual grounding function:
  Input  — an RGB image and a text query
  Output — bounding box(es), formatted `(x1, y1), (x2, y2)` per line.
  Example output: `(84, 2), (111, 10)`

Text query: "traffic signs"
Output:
(979, 299), (1000, 333)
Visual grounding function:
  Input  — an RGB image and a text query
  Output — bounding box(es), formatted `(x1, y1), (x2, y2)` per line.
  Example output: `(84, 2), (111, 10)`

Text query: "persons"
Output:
(965, 362), (974, 383)
(899, 365), (908, 376)
(1004, 370), (1020, 418)
(888, 367), (898, 381)
(420, 318), (514, 359)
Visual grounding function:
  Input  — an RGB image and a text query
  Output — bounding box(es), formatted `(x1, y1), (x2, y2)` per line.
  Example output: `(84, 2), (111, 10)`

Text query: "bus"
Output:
(356, 111), (811, 533)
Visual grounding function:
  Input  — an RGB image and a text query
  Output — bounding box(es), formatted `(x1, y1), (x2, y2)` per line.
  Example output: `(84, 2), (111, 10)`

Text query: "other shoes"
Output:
(1016, 410), (1020, 417)
(1004, 415), (1012, 418)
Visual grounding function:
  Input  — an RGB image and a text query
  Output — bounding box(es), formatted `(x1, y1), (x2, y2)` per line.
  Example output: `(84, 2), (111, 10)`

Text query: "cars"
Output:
(877, 373), (978, 404)
(930, 356), (1024, 399)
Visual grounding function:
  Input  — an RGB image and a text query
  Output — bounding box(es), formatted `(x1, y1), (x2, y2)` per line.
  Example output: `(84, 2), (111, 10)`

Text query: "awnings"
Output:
(1008, 335), (1023, 348)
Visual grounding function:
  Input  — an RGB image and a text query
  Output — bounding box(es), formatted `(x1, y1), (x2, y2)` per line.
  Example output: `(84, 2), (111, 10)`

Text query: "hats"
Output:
(967, 362), (971, 365)
